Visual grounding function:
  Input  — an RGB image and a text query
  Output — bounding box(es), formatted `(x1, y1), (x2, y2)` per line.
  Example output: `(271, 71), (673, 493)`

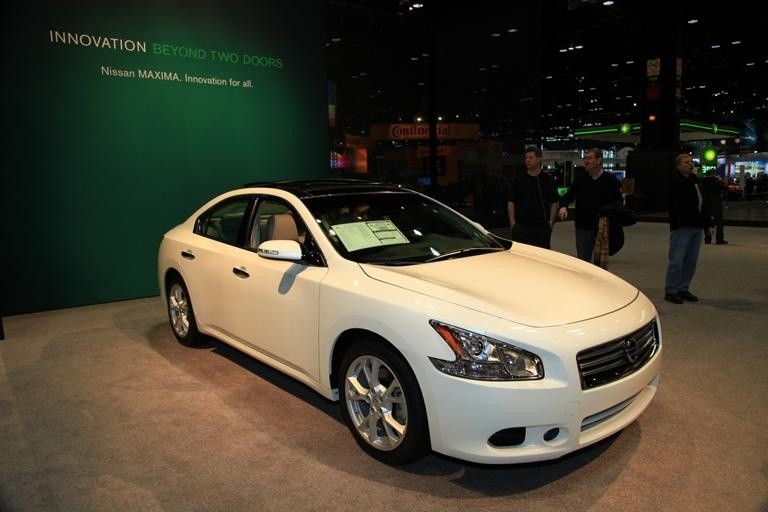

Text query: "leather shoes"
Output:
(716, 239), (728, 244)
(665, 291), (699, 304)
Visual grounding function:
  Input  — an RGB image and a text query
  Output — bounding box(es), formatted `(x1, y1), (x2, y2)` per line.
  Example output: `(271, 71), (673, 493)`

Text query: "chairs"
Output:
(221, 211), (302, 240)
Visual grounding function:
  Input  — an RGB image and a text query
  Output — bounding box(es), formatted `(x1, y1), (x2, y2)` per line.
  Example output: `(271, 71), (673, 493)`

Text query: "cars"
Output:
(156, 177), (663, 470)
(540, 173), (577, 206)
(698, 176), (743, 201)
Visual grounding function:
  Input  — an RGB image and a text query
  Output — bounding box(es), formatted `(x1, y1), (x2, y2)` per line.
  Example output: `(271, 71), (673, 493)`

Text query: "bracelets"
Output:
(549, 221), (554, 225)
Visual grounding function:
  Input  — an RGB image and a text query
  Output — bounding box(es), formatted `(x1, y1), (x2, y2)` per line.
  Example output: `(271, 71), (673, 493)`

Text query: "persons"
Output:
(558, 147), (623, 270)
(507, 147), (560, 249)
(701, 170), (728, 244)
(665, 153), (715, 304)
